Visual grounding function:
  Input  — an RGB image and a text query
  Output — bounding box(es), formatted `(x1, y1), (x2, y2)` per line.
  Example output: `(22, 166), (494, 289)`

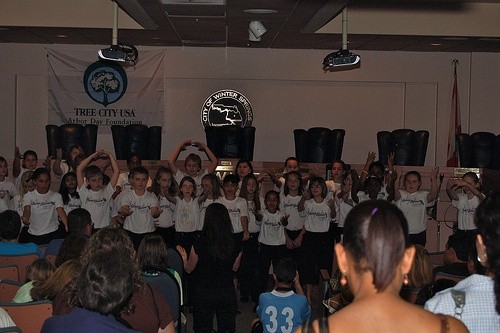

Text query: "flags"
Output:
(446, 66), (462, 168)
(47, 47), (165, 134)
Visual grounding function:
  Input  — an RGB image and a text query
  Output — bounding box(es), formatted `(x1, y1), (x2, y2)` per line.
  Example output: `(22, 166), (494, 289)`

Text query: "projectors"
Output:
(97, 49), (132, 63)
(328, 55), (360, 67)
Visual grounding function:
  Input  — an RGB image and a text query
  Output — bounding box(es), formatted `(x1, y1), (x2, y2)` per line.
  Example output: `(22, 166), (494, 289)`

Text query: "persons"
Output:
(57, 233), (91, 267)
(30, 258), (83, 302)
(11, 258), (56, 303)
(446, 172), (487, 275)
(251, 261), (312, 333)
(40, 252), (145, 333)
(434, 233), (469, 277)
(0, 210), (45, 259)
(136, 233), (188, 333)
(52, 225), (176, 333)
(176, 203), (243, 333)
(0, 144), (120, 245)
(252, 152), (439, 312)
(45, 208), (94, 259)
(466, 241), (485, 275)
(111, 153), (266, 314)
(294, 199), (471, 333)
(168, 139), (219, 199)
(424, 192), (500, 333)
(0, 307), (18, 333)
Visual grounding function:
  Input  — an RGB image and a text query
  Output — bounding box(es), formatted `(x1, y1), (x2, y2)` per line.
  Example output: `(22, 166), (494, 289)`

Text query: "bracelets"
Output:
(15, 156), (20, 159)
(362, 170), (368, 175)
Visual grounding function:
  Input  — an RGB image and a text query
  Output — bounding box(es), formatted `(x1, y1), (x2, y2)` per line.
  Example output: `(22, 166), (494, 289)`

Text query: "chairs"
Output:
(47, 124), (99, 159)
(319, 251), (466, 333)
(456, 131), (500, 170)
(293, 127), (346, 163)
(111, 124), (163, 160)
(0, 237), (187, 333)
(205, 126), (256, 161)
(377, 129), (429, 166)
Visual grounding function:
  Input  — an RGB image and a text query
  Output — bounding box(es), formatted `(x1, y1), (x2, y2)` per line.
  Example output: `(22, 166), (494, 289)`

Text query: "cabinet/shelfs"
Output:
(400, 172), (464, 250)
